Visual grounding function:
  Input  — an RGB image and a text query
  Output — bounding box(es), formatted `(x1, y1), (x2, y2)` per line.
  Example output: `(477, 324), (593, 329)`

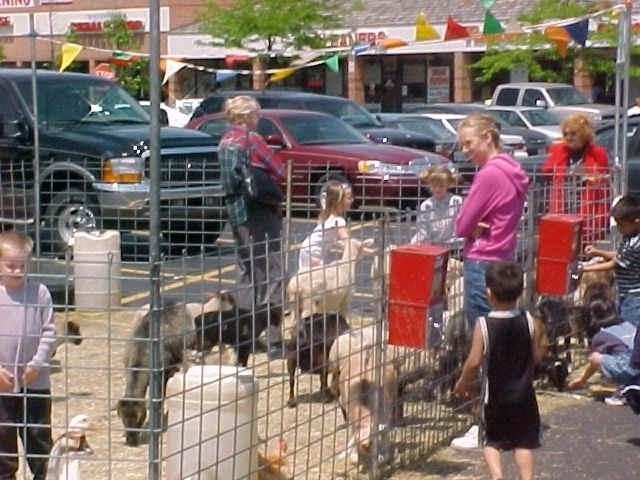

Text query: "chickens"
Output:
(258, 438), (291, 480)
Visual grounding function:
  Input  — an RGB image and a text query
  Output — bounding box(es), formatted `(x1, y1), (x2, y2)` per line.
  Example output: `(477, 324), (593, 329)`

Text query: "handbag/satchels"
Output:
(241, 167), (283, 205)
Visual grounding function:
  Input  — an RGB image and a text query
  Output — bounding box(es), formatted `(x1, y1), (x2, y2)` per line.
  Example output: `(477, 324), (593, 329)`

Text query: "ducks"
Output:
(59, 414), (104, 480)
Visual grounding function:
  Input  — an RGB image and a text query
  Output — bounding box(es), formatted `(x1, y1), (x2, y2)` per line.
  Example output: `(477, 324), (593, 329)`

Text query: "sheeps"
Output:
(533, 255), (620, 394)
(287, 311), (469, 468)
(109, 295), (194, 447)
(194, 302), (283, 366)
(287, 237), (378, 320)
(132, 288), (237, 355)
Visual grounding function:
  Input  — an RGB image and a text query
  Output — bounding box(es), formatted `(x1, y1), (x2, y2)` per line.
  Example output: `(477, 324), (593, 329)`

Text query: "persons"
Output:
(454, 260), (542, 480)
(627, 96), (640, 116)
(541, 114), (610, 273)
(218, 95), (285, 313)
(1, 231), (57, 480)
(298, 181), (377, 272)
(563, 297), (640, 405)
(577, 193), (640, 324)
(415, 166), (465, 278)
(454, 112), (529, 377)
(620, 323), (639, 418)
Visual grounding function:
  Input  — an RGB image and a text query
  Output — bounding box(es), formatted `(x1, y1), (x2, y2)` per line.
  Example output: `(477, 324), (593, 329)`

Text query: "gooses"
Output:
(45, 432), (94, 480)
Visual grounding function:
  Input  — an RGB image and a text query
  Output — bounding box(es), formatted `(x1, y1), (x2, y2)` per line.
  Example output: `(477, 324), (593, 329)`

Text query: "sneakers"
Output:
(605, 390), (627, 405)
(451, 425), (480, 451)
